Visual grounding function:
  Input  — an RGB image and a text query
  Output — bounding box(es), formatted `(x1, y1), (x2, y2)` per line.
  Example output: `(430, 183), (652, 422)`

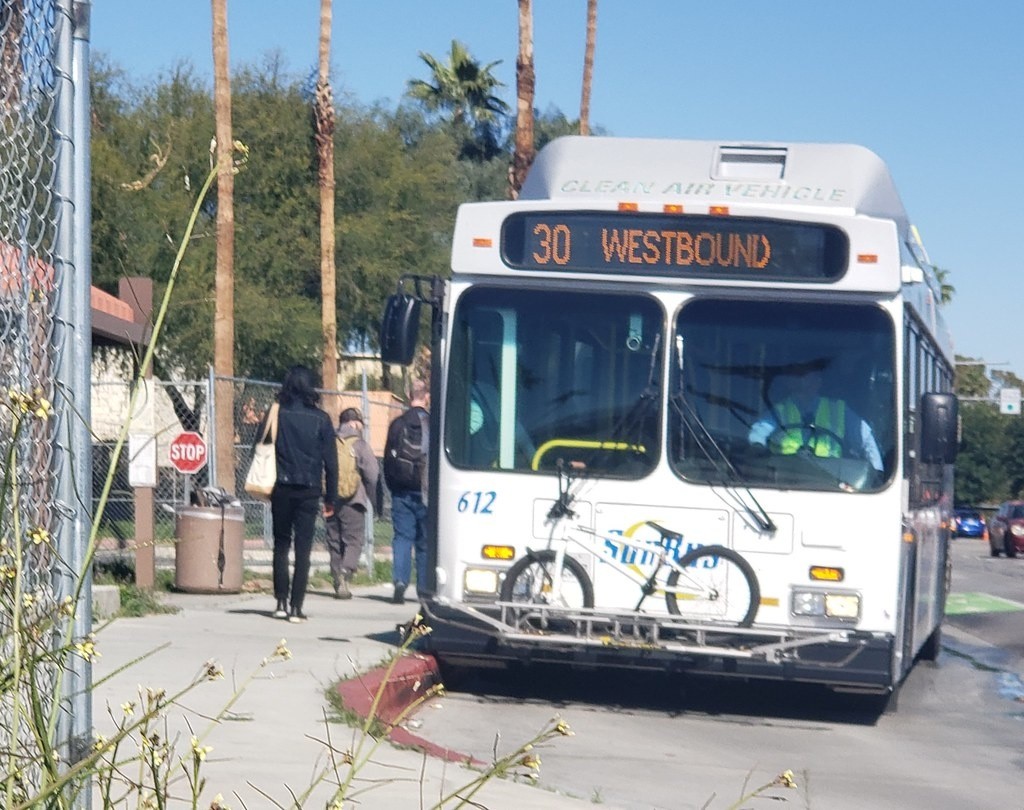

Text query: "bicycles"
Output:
(495, 456), (763, 654)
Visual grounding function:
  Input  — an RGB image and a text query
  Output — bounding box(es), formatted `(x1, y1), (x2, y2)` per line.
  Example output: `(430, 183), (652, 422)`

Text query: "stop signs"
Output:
(168, 434), (207, 476)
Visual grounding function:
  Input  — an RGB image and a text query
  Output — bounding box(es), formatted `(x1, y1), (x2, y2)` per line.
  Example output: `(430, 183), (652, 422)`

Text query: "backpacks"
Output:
(390, 412), (426, 493)
(321, 435), (362, 502)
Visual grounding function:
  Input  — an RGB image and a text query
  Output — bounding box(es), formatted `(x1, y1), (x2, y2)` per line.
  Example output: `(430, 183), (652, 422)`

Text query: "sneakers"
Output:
(392, 582), (406, 604)
(288, 606), (308, 624)
(418, 592), (433, 604)
(272, 600), (289, 620)
(332, 576), (351, 600)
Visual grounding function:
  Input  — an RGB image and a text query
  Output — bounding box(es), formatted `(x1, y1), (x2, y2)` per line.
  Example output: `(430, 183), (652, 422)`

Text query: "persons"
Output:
(382, 380), (431, 605)
(736, 351), (883, 472)
(248, 364), (339, 625)
(321, 406), (385, 598)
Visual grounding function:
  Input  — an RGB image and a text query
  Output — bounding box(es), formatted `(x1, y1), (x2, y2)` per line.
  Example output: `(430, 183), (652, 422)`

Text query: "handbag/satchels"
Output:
(244, 402), (282, 503)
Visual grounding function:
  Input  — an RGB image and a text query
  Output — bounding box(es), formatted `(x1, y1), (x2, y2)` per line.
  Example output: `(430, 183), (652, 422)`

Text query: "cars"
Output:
(985, 498), (1024, 558)
(952, 510), (987, 538)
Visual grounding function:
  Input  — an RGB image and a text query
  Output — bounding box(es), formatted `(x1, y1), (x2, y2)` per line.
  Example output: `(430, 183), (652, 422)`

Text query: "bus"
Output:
(730, 538), (739, 542)
(378, 131), (971, 713)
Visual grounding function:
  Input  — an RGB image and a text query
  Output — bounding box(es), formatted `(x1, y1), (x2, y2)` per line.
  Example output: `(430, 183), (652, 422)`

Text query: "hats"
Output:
(339, 408), (364, 426)
